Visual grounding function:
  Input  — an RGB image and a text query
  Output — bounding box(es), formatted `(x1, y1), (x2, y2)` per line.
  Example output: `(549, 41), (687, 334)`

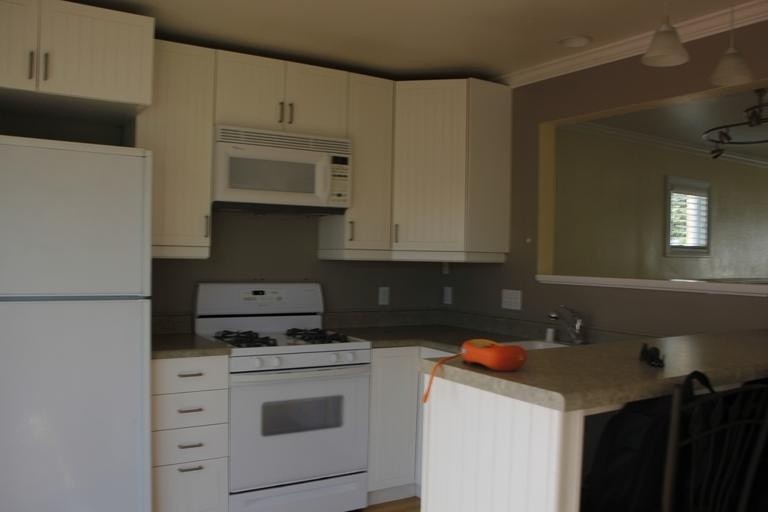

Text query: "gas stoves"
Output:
(196, 315), (372, 372)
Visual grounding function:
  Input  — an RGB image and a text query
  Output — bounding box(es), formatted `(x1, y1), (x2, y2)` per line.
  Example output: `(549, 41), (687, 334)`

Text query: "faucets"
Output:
(547, 304), (590, 346)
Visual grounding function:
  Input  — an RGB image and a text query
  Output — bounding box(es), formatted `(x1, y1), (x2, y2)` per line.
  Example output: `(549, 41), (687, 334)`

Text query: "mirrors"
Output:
(534, 74), (767, 301)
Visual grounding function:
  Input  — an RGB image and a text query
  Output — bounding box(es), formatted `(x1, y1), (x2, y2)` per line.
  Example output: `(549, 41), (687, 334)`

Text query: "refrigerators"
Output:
(0, 134), (155, 510)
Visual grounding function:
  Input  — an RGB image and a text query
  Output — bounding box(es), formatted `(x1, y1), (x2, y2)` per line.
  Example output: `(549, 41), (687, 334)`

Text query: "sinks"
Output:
(498, 339), (571, 352)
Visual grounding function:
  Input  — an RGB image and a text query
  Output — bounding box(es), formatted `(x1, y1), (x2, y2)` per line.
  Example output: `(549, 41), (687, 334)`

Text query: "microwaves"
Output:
(215, 127), (351, 214)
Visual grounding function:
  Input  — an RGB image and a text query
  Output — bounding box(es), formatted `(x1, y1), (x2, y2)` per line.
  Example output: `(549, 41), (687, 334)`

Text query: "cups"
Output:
(545, 326), (558, 344)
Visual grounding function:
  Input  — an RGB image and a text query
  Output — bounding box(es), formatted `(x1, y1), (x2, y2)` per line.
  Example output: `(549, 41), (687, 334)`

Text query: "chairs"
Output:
(648, 375), (767, 511)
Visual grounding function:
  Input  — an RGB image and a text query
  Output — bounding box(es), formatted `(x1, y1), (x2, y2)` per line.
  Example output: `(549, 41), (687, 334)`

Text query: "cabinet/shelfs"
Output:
(367, 342), (417, 504)
(127, 35), (216, 262)
(412, 346), (455, 502)
(392, 72), (513, 267)
(213, 44), (351, 141)
(151, 351), (233, 512)
(1, 0), (156, 119)
(318, 71), (395, 264)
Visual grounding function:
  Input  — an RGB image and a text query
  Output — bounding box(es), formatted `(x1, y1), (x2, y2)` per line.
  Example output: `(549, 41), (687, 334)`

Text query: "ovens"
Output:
(227, 365), (369, 510)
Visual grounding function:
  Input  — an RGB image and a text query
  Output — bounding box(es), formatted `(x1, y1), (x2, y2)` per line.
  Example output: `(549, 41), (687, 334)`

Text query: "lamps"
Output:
(638, 0), (691, 69)
(708, 1), (756, 88)
